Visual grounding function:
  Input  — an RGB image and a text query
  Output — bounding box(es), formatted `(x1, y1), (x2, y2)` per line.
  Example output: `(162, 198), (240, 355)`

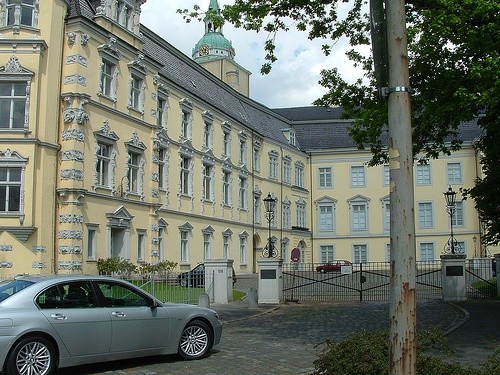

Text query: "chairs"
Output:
(69, 287), (112, 308)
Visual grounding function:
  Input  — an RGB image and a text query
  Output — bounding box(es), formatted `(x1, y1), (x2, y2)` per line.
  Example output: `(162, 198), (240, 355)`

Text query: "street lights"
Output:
(261, 191), (279, 258)
(442, 184), (462, 255)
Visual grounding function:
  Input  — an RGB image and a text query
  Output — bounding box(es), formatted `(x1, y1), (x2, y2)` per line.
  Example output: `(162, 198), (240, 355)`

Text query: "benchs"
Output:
(45, 299), (64, 308)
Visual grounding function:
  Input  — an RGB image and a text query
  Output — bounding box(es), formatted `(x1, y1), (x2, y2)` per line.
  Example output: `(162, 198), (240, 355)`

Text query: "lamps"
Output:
(262, 192), (279, 259)
(442, 185), (462, 254)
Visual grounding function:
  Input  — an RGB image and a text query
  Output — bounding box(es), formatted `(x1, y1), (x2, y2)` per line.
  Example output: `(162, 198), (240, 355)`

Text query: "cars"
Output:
(0, 274), (223, 375)
(177, 263), (237, 290)
(315, 259), (353, 273)
